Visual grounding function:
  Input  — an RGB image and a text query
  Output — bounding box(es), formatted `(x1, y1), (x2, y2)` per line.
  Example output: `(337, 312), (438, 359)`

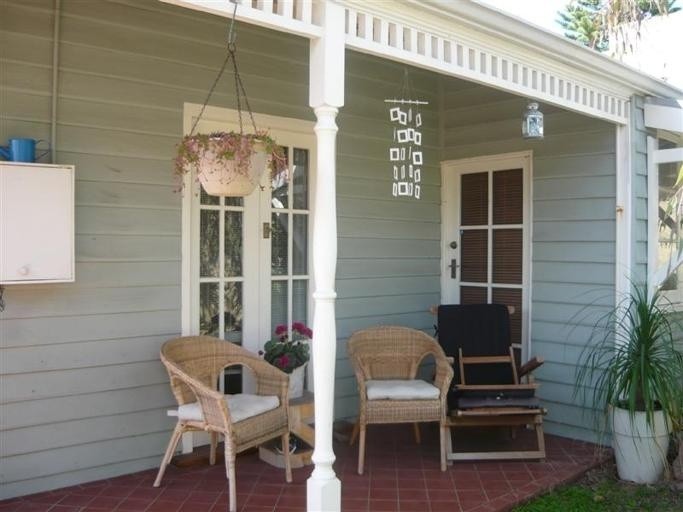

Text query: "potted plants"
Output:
(560, 261), (683, 485)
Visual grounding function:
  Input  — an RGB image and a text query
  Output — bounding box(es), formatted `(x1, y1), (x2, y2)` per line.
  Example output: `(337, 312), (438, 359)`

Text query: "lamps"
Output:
(520, 101), (545, 139)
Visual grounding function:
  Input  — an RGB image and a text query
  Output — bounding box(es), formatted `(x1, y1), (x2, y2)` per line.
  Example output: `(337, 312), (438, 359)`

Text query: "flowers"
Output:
(258, 321), (313, 368)
(170, 134), (291, 183)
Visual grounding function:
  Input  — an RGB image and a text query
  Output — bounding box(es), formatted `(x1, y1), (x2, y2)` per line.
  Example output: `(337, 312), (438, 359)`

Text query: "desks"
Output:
(258, 390), (315, 483)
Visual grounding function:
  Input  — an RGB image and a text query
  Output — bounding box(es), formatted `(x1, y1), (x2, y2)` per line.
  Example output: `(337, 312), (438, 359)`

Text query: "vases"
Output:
(284, 361), (309, 398)
(191, 139), (268, 195)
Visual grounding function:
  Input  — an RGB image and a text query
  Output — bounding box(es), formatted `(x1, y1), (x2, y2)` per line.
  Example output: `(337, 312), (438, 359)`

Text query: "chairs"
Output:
(151, 335), (293, 512)
(348, 324), (455, 475)
(430, 303), (548, 468)
